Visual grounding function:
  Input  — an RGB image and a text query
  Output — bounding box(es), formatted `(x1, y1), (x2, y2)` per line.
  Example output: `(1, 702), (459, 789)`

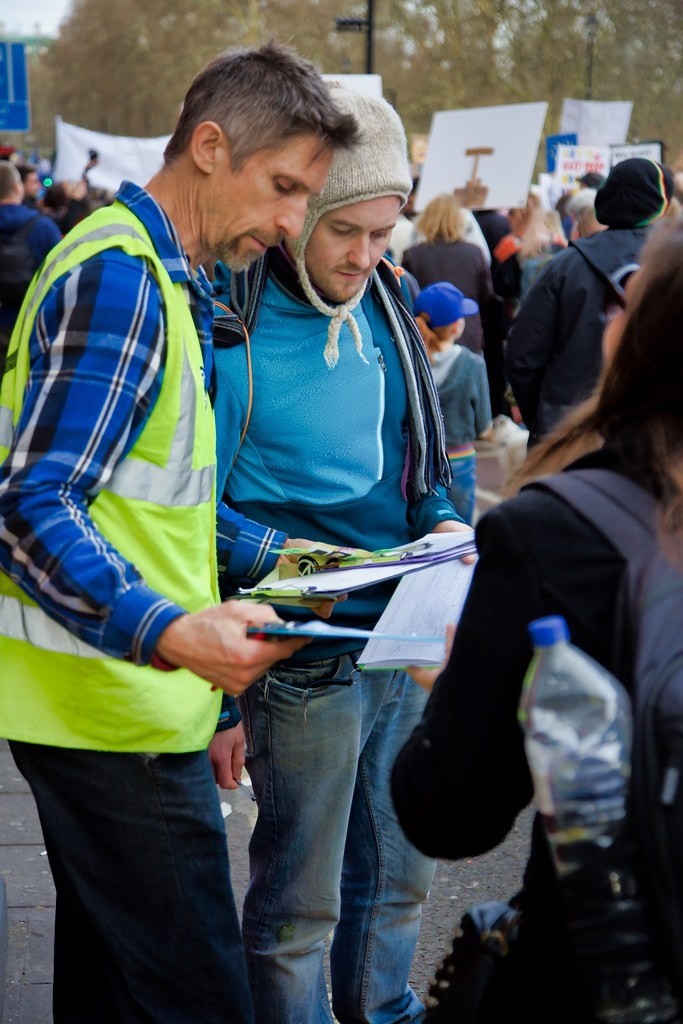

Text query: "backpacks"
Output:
(519, 467), (682, 1024)
(0, 213), (43, 324)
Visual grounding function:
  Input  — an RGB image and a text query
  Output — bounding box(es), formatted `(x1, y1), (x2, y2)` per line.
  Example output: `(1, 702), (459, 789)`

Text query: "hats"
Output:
(594, 158), (674, 229)
(413, 282), (479, 328)
(284, 80), (413, 367)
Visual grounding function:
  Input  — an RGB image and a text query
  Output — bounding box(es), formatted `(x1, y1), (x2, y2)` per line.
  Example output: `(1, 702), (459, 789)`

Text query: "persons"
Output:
(385, 172), (610, 528)
(211, 87), (474, 1024)
(388, 195), (682, 1024)
(0, 142), (119, 383)
(0, 47), (361, 1024)
(499, 158), (683, 459)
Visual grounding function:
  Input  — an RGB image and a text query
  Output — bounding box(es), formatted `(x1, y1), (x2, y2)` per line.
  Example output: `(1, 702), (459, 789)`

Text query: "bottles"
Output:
(517, 615), (632, 880)
